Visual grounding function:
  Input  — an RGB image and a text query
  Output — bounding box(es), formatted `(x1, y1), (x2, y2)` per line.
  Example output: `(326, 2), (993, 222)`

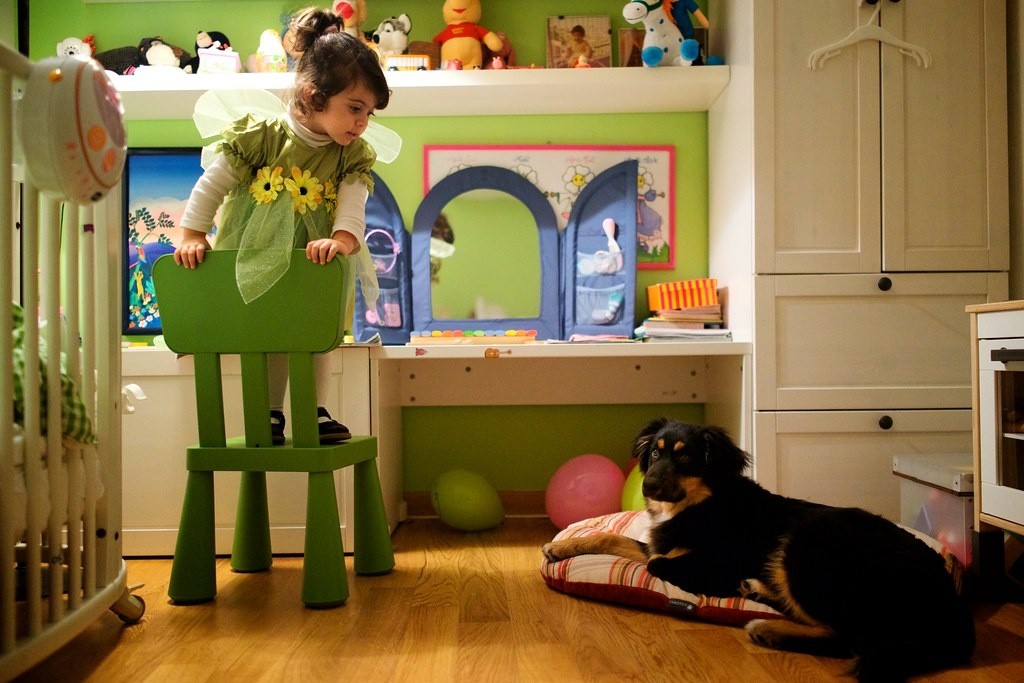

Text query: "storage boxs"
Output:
(648, 279), (719, 312)
(892, 453), (974, 569)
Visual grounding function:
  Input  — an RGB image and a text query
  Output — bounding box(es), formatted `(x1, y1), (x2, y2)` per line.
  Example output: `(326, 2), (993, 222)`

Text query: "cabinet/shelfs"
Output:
(963, 300), (1024, 577)
(710, 0), (1010, 528)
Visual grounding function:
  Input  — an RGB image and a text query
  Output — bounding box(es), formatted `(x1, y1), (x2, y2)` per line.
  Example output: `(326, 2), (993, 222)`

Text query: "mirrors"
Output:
(430, 188), (541, 321)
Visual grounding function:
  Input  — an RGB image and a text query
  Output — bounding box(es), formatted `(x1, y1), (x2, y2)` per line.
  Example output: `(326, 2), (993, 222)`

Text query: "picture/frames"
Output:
(423, 145), (675, 269)
(121, 147), (221, 336)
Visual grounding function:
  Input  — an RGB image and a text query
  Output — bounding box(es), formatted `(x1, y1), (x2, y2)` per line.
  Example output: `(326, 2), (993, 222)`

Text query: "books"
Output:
(634, 305), (734, 342)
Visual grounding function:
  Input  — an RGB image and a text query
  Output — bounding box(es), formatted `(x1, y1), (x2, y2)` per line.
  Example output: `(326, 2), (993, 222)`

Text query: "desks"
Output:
(45, 342), (753, 555)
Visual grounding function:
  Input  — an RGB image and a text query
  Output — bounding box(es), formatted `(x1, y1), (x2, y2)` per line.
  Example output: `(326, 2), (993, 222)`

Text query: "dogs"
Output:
(540, 413), (1004, 683)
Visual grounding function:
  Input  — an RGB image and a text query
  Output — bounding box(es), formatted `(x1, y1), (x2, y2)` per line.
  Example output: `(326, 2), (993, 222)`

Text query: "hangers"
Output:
(808, 0), (932, 71)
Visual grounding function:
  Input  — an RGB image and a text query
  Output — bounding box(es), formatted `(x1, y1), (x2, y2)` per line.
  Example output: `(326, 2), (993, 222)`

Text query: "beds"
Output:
(0, 44), (145, 683)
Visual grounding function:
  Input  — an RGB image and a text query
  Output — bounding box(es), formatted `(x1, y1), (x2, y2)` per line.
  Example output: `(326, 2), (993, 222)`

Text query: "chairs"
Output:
(152, 248), (395, 607)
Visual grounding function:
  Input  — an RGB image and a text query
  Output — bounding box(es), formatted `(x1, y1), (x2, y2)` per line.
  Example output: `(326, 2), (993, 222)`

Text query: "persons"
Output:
(174, 7), (403, 444)
(563, 25), (593, 67)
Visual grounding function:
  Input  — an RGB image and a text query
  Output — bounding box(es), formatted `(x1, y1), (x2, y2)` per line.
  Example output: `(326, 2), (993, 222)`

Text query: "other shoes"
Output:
(269, 410), (286, 444)
(316, 406), (351, 442)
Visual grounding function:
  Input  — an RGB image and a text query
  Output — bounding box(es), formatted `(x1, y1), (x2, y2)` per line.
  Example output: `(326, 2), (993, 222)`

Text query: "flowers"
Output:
(248, 166), (336, 214)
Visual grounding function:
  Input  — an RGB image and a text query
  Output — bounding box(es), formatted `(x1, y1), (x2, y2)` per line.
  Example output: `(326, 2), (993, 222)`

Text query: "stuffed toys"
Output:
(279, 0), (515, 71)
(97, 31), (231, 74)
(622, 0), (726, 68)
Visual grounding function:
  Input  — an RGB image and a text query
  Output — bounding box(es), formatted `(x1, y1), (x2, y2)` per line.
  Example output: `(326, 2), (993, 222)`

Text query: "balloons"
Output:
(431, 468), (505, 530)
(546, 453), (647, 530)
